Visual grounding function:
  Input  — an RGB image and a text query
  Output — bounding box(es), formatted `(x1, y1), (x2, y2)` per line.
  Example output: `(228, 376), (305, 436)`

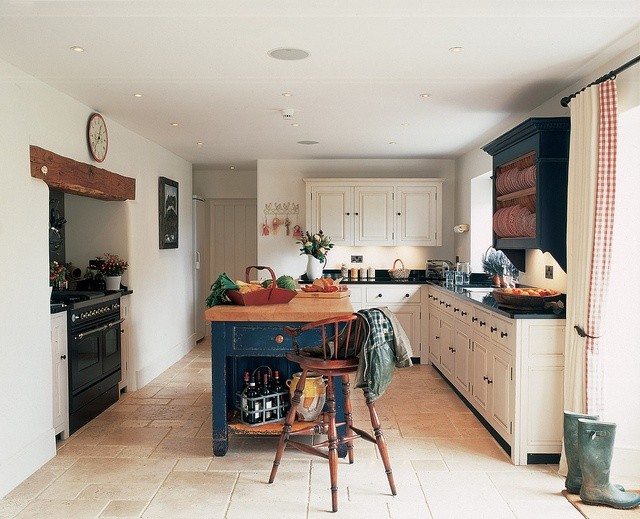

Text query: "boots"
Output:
(561, 410), (624, 495)
(577, 418), (640, 509)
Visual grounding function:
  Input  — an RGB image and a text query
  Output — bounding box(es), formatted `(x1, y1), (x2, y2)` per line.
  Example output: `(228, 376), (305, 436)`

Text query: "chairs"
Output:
(269, 314), (395, 511)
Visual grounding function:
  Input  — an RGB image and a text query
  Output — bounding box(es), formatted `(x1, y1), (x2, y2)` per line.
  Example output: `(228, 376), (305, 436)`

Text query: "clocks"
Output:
(86, 113), (108, 163)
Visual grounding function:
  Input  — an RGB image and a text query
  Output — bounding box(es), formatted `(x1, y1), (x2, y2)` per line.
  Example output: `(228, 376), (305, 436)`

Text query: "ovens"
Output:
(68, 314), (125, 413)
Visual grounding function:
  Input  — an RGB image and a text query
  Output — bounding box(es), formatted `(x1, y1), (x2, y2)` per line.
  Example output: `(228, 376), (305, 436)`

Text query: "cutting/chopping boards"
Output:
(295, 290), (350, 299)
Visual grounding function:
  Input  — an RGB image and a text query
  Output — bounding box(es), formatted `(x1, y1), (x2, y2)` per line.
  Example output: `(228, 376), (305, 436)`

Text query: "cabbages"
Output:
(276, 275), (296, 292)
(262, 279), (273, 288)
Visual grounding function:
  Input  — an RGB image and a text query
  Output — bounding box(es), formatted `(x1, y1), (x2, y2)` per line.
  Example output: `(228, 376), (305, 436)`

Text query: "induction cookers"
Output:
(51, 290), (122, 328)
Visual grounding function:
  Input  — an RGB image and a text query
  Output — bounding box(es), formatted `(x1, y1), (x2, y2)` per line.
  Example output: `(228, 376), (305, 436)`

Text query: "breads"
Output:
(303, 277), (348, 292)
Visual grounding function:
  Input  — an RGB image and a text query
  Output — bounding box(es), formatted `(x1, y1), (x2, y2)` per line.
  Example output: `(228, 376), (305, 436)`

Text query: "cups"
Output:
(455, 271), (463, 286)
(446, 270), (454, 288)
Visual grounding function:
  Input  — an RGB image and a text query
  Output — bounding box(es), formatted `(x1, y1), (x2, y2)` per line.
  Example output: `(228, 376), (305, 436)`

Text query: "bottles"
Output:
(248, 372), (259, 424)
(240, 372), (249, 422)
(259, 374), (272, 421)
(256, 371), (263, 392)
(272, 370), (283, 418)
(267, 368), (273, 392)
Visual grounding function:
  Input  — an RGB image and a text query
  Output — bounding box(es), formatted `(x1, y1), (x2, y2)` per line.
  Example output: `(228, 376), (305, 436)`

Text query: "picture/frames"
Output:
(158, 176), (179, 249)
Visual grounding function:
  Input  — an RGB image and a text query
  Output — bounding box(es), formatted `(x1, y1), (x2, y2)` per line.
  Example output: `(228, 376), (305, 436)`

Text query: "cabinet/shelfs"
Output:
(52, 311), (69, 439)
(366, 285), (421, 358)
(297, 283), (361, 314)
(441, 292), (471, 402)
(428, 284), (444, 374)
(472, 304), (513, 447)
(480, 116), (569, 273)
(395, 177), (442, 246)
(306, 179), (395, 246)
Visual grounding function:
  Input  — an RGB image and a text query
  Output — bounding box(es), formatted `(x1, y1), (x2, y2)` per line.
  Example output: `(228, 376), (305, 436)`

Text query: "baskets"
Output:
(225, 266), (298, 306)
(388, 259), (411, 279)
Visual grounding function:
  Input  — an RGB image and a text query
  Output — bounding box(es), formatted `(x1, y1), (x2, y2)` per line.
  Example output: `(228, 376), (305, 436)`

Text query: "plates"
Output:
(492, 289), (561, 307)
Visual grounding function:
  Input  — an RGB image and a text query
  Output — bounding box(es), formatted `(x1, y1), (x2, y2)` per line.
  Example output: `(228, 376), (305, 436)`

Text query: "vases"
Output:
(285, 371), (332, 425)
(306, 255), (321, 281)
(104, 276), (121, 291)
(49, 287), (53, 303)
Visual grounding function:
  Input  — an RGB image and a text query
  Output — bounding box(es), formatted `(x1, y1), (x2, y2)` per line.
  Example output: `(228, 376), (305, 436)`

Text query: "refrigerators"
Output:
(192, 194), (206, 341)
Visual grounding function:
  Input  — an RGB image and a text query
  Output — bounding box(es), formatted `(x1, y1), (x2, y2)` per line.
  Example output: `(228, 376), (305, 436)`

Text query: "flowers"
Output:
(295, 229), (335, 270)
(95, 252), (130, 276)
(50, 257), (66, 287)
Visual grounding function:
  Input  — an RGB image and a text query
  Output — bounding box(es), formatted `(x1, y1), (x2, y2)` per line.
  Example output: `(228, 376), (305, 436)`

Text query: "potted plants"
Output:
(509, 262), (519, 289)
(500, 252), (511, 288)
(482, 249), (501, 288)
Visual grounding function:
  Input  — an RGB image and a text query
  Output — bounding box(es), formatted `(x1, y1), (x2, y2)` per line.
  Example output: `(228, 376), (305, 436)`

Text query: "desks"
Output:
(201, 286), (355, 459)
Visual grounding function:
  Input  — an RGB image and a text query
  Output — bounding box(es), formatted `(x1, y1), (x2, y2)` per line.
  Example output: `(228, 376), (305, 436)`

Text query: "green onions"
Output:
(205, 271), (239, 309)
